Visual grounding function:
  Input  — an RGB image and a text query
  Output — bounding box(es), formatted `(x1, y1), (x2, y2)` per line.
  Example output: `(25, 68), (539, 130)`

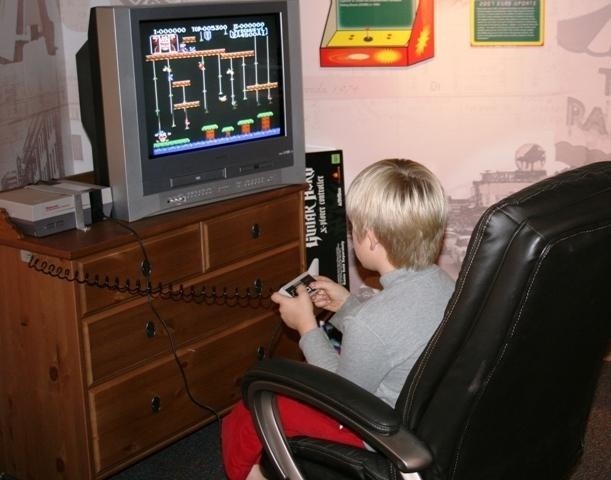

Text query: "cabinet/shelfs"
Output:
(0, 183), (305, 480)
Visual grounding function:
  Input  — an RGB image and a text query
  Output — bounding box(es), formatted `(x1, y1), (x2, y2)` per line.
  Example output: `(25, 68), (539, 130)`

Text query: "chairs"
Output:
(242, 159), (610, 479)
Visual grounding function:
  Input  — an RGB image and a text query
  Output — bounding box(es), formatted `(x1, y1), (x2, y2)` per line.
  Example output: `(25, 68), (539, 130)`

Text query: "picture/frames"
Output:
(470, 0), (545, 46)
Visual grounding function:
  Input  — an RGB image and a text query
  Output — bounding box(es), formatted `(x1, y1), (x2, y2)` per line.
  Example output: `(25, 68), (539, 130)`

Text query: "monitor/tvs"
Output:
(75, 0), (306, 225)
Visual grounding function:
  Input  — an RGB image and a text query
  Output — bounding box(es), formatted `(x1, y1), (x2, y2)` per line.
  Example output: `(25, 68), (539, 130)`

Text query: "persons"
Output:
(221, 158), (458, 480)
